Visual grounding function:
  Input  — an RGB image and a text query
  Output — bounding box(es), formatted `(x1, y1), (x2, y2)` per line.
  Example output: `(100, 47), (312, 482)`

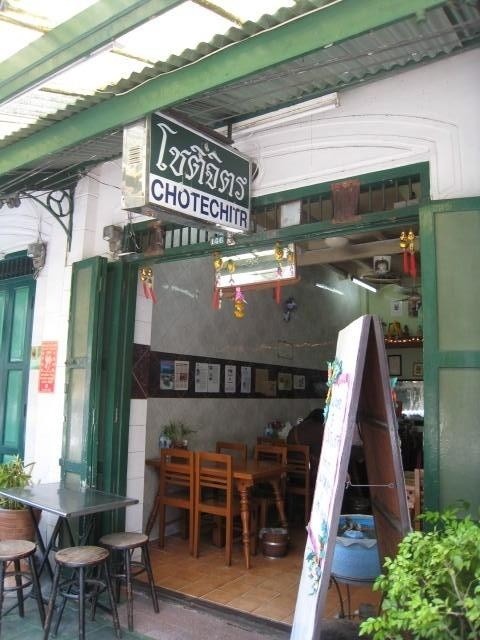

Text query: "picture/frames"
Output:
(412, 362), (423, 377)
(387, 355), (402, 376)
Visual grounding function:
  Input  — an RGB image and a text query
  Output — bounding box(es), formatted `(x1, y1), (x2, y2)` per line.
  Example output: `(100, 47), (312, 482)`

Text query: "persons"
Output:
(286, 408), (324, 486)
(350, 421), (363, 495)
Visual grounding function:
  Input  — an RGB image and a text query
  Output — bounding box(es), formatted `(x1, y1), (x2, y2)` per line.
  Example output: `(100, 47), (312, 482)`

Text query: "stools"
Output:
(90, 532), (160, 632)
(42, 545), (121, 640)
(0, 539), (46, 630)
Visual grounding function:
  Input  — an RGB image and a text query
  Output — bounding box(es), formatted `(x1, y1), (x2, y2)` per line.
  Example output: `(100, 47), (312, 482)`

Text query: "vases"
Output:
(330, 514), (381, 581)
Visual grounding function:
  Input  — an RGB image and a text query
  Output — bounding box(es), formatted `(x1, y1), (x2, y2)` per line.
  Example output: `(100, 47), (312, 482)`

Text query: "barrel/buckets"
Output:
(259, 527), (290, 560)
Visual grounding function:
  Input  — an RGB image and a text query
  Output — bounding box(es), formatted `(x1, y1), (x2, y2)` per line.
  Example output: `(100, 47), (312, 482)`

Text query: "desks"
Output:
(146, 458), (295, 568)
(0, 482), (140, 635)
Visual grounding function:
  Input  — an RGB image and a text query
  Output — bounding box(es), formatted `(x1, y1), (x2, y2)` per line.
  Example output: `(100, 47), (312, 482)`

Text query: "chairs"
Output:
(194, 451), (257, 566)
(207, 441), (247, 498)
(279, 445), (313, 526)
(414, 467), (424, 531)
(251, 445), (287, 528)
(158, 448), (193, 555)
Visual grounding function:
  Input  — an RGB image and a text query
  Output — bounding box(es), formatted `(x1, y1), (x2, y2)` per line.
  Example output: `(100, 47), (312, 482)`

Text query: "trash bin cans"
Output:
(262, 527), (288, 560)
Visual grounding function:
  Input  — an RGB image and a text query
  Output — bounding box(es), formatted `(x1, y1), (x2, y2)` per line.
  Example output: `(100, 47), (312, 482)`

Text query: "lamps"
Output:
(351, 276), (378, 293)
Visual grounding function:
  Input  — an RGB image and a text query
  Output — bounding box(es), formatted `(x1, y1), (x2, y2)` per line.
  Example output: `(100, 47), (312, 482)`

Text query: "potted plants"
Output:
(0, 454), (41, 573)
(161, 422), (197, 460)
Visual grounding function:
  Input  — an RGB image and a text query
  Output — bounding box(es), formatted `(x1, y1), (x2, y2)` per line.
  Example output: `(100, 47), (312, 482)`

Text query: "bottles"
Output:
(403, 325), (408, 336)
(159, 432), (175, 459)
(264, 423), (273, 442)
(415, 324), (423, 338)
